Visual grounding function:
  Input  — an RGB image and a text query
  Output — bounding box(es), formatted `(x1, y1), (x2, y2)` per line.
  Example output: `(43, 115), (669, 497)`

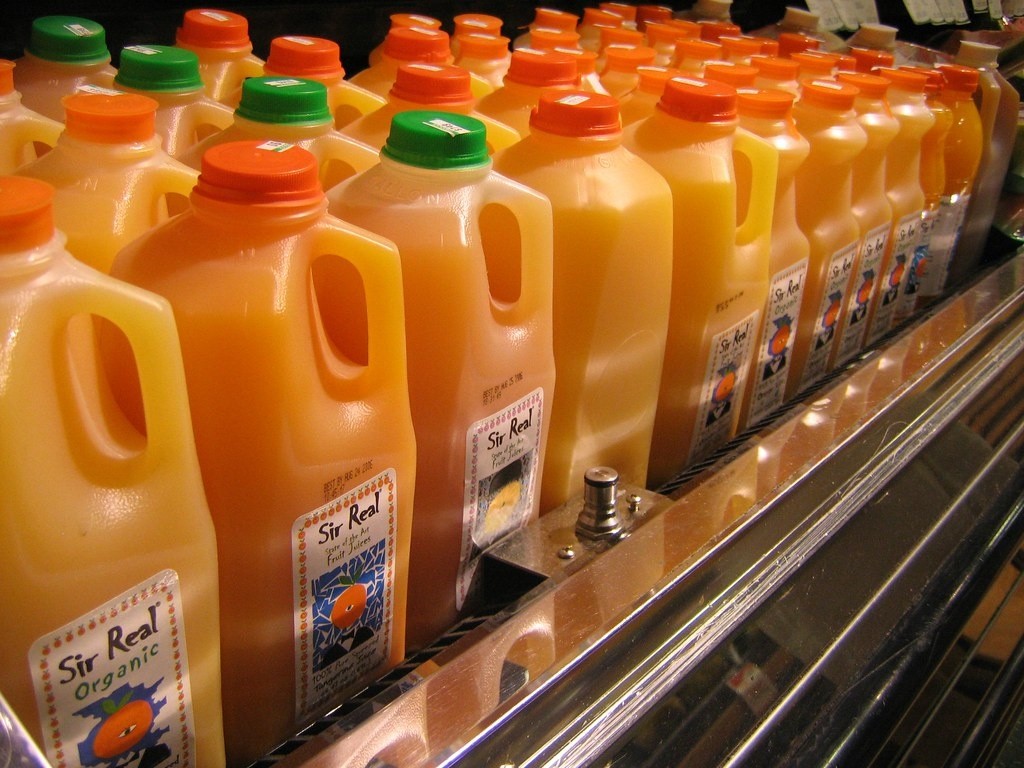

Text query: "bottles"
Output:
(1, 4), (1021, 768)
